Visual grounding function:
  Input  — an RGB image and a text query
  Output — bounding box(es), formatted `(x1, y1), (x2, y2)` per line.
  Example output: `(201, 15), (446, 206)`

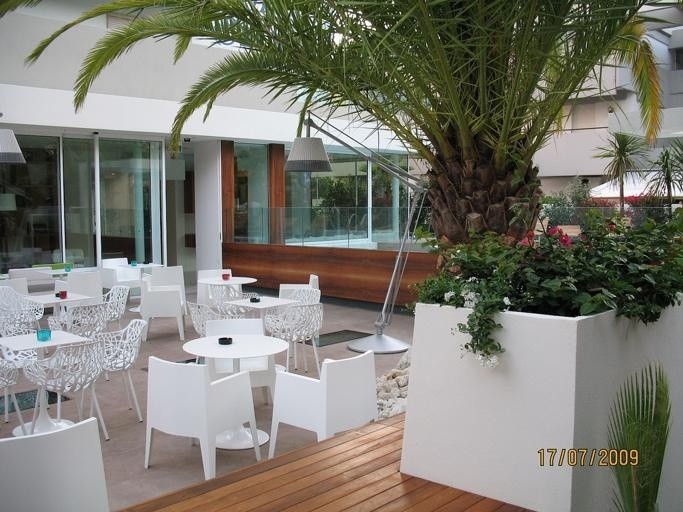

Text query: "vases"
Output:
(399, 292), (683, 511)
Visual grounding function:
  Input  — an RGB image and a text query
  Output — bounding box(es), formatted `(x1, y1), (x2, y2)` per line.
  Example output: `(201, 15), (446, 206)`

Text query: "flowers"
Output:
(403, 208), (683, 367)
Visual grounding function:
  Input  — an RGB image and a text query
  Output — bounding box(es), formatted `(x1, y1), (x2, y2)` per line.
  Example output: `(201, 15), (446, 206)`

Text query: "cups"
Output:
(36, 328), (51, 341)
(64, 263), (69, 272)
(130, 259), (137, 266)
(58, 290), (66, 299)
(221, 273), (230, 281)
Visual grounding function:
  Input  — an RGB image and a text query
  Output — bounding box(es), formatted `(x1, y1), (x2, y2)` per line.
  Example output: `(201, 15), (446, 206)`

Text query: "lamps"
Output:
(0, 129), (27, 164)
(0, 192), (17, 211)
(283, 109), (430, 355)
